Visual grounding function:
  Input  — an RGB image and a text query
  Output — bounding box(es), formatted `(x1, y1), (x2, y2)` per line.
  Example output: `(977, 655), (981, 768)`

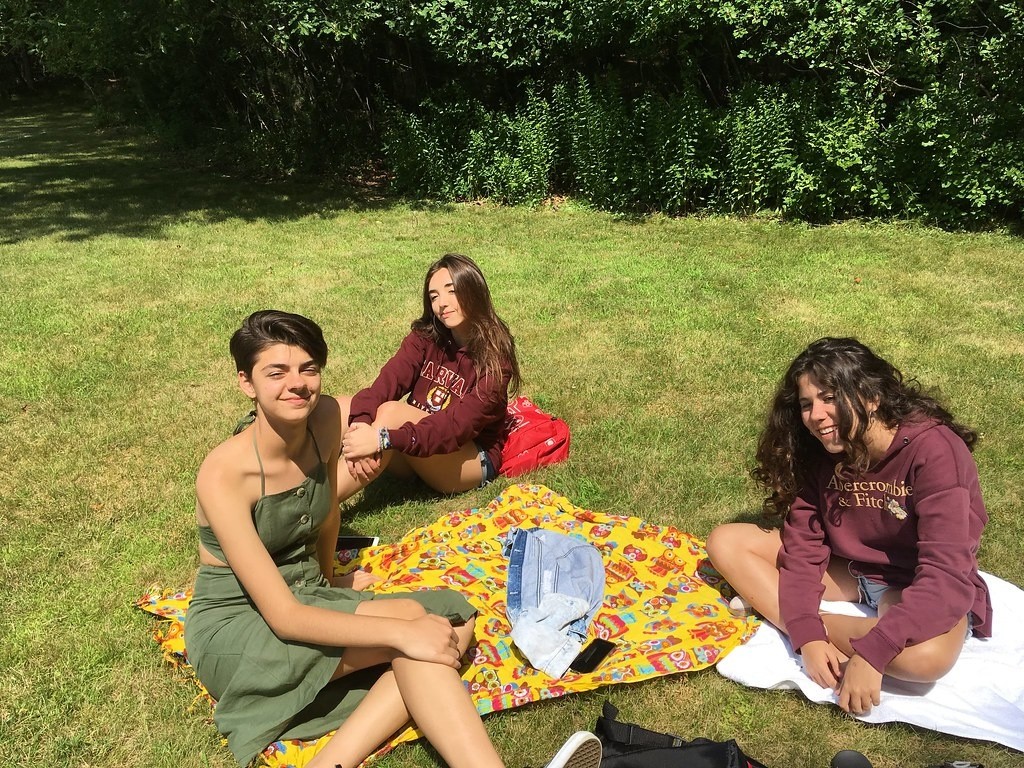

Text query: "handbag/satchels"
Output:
(594, 702), (767, 768)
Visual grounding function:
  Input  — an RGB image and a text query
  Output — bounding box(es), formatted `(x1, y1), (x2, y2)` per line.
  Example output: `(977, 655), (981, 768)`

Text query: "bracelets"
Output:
(377, 427), (389, 452)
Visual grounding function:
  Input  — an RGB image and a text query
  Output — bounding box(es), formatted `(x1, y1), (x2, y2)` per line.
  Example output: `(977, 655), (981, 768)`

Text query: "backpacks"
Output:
(487, 395), (571, 478)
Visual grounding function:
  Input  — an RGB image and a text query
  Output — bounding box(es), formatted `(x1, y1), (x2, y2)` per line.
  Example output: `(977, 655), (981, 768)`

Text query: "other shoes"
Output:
(542, 731), (602, 768)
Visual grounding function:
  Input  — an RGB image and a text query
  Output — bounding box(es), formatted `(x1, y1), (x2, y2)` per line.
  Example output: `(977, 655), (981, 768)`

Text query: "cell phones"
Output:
(335, 535), (380, 553)
(569, 638), (616, 673)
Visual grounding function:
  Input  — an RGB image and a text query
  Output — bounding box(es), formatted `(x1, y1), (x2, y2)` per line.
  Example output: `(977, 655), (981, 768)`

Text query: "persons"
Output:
(182, 308), (603, 768)
(327, 251), (514, 507)
(704, 337), (992, 715)
